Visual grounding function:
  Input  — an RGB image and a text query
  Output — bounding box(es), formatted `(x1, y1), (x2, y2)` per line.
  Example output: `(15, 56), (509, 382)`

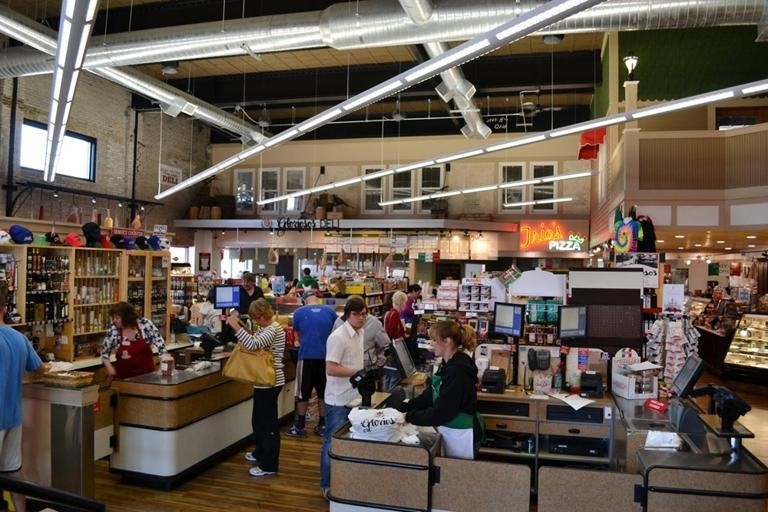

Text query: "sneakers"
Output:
(285, 425), (307, 437)
(245, 452), (257, 461)
(249, 466), (275, 476)
(314, 425), (326, 436)
(322, 488), (331, 501)
(305, 412), (311, 421)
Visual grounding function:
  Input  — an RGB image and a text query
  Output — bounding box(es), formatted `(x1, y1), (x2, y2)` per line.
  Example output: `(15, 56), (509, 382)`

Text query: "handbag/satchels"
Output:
(222, 343), (276, 386)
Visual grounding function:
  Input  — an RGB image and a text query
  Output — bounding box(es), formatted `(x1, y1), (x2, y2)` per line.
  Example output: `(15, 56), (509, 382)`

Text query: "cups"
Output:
(161, 357), (174, 377)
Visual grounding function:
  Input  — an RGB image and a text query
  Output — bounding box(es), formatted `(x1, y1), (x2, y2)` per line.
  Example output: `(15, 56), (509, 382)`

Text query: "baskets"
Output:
(43, 371), (94, 388)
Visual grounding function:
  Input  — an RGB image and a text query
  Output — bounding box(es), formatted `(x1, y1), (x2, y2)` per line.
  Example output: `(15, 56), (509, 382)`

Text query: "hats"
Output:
(0, 225), (33, 244)
(34, 222), (170, 249)
(302, 289), (322, 305)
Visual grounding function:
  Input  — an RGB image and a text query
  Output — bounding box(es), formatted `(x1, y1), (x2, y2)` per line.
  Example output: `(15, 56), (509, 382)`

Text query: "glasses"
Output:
(252, 314), (263, 321)
(350, 312), (367, 317)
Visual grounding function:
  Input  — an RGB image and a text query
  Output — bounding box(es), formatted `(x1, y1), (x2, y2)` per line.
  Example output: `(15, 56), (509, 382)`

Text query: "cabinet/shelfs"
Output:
(683, 296), (711, 326)
(724, 312), (768, 395)
(345, 277), (409, 320)
(170, 275), (199, 332)
(475, 384), (615, 493)
(71, 247), (125, 360)
(414, 294), (506, 373)
(126, 250), (171, 345)
(0, 244), (73, 362)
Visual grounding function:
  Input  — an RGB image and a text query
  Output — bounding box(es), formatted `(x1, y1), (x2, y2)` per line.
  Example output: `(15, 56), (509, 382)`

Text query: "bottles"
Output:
(305, 409), (311, 421)
(128, 282), (167, 328)
(169, 276), (197, 308)
(528, 371), (533, 391)
(39, 205), (141, 229)
(527, 438), (532, 454)
(554, 368), (562, 393)
(171, 328), (176, 343)
(699, 312), (705, 326)
(128, 256), (167, 277)
(228, 306), (241, 318)
(74, 249), (120, 333)
(25, 247), (69, 320)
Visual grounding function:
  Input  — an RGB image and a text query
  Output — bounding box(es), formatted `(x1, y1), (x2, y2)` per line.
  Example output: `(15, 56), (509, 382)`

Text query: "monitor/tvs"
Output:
(389, 336), (417, 379)
(556, 303), (589, 339)
(492, 301), (526, 339)
(671, 353), (705, 399)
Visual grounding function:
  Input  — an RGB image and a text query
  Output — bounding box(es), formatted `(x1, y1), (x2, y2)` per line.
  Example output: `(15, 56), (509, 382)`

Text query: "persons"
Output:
(101, 301), (167, 379)
(226, 297), (286, 477)
(397, 320), (487, 460)
(0, 291), (46, 512)
(319, 297), (369, 501)
(198, 268), (422, 366)
(285, 289), (338, 437)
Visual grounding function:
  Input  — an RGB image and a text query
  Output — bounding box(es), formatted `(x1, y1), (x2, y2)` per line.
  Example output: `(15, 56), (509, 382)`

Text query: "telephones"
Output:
(527, 348), (550, 371)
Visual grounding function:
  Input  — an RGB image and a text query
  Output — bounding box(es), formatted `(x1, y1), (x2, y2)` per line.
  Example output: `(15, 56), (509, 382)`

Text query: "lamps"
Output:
(154, 0), (607, 201)
(43, 1), (102, 182)
(377, 35), (599, 208)
(257, 0), (767, 206)
(622, 50), (639, 81)
(503, 87), (576, 204)
